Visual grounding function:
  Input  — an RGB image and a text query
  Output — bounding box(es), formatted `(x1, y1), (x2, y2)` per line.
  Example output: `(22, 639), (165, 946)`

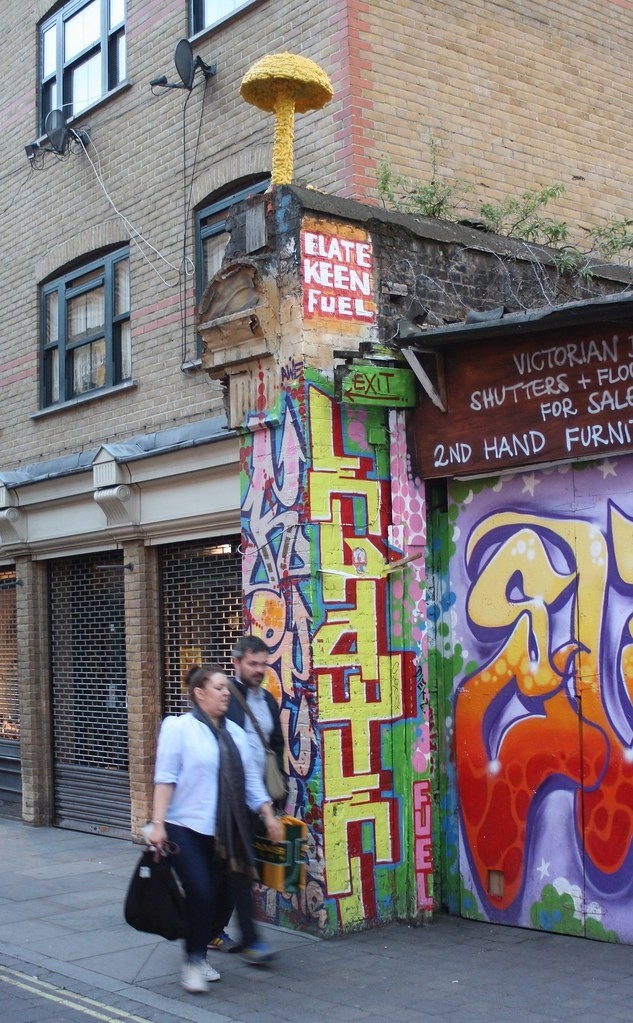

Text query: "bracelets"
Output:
(151, 820), (163, 823)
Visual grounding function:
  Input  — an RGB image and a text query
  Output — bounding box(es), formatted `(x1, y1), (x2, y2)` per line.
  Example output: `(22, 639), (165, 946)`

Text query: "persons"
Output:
(150, 665), (283, 991)
(205, 636), (286, 963)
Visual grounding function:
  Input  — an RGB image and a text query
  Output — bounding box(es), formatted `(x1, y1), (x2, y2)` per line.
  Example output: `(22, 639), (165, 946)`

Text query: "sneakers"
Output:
(208, 932), (241, 951)
(242, 945), (271, 964)
(184, 966), (209, 992)
(199, 961), (221, 981)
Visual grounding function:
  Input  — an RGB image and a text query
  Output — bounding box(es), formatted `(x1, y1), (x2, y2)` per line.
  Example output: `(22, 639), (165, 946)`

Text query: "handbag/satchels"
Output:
(125, 841), (190, 940)
(266, 750), (288, 800)
